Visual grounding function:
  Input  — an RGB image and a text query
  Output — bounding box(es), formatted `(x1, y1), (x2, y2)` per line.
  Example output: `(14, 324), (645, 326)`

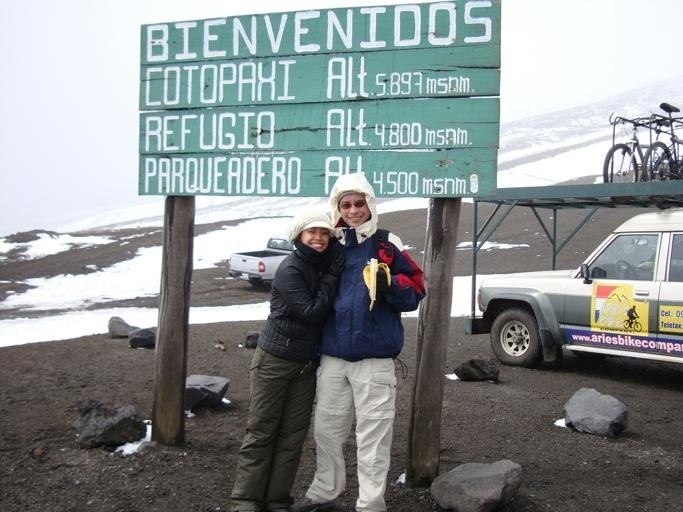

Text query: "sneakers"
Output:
(290, 495), (336, 512)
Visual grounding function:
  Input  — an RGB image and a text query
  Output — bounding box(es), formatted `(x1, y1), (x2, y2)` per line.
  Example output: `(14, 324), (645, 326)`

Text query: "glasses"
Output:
(342, 200), (366, 209)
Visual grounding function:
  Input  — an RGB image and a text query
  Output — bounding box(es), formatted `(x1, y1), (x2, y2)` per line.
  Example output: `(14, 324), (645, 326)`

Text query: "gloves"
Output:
(376, 270), (398, 292)
(326, 252), (344, 277)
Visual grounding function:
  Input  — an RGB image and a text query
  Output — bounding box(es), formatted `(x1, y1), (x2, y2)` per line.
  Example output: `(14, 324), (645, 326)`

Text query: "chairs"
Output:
(670, 240), (683, 281)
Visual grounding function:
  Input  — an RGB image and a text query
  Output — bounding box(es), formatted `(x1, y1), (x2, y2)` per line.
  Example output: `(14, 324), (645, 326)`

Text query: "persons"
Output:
(229, 208), (347, 511)
(297, 173), (426, 512)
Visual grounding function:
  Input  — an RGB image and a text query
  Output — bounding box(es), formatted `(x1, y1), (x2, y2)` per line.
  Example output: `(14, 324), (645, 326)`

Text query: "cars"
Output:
(477, 207), (682, 366)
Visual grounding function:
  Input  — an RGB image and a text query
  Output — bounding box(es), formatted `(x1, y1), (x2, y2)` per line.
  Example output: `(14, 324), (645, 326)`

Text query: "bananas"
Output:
(363, 259), (391, 311)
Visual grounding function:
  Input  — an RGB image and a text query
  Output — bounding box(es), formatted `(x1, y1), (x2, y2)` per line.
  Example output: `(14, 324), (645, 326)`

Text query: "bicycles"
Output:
(602, 103), (683, 183)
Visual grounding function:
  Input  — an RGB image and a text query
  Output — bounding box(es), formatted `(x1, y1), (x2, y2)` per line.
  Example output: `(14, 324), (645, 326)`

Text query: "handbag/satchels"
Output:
(454, 359), (499, 381)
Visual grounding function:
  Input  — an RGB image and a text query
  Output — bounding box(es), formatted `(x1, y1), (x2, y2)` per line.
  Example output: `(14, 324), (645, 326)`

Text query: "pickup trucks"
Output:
(229, 237), (297, 285)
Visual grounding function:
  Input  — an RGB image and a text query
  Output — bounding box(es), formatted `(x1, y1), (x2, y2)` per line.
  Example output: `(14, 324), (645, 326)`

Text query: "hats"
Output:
(289, 210), (334, 246)
(337, 191), (364, 211)
